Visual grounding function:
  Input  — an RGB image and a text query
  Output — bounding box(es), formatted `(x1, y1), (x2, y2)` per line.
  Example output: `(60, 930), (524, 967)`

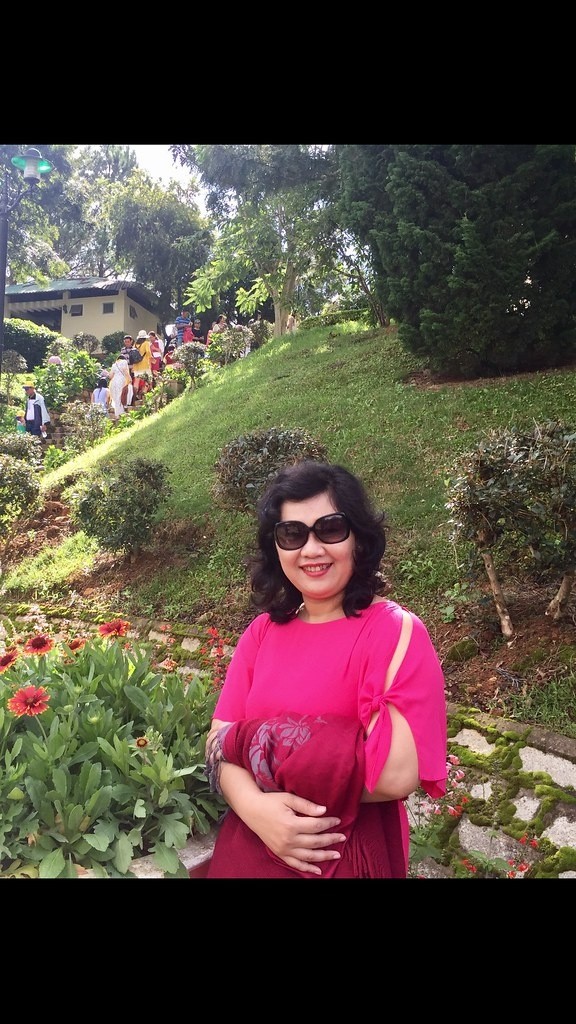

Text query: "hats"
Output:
(194, 319), (201, 323)
(22, 380), (36, 388)
(137, 330), (150, 339)
(15, 410), (26, 417)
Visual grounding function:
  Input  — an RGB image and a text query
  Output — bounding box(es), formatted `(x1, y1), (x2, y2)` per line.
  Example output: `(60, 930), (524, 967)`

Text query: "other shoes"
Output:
(135, 394), (140, 400)
(142, 392), (147, 396)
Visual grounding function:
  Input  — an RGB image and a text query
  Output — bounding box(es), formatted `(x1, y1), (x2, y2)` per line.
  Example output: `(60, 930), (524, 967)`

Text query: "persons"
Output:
(87, 309), (262, 424)
(205, 463), (447, 879)
(14, 385), (50, 438)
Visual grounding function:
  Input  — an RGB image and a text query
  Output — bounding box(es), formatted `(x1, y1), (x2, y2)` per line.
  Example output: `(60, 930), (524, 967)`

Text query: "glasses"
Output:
(273, 511), (351, 551)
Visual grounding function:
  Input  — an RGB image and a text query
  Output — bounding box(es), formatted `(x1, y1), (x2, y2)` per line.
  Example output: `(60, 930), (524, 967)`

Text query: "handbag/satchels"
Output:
(152, 351), (161, 358)
(183, 327), (194, 343)
(127, 378), (131, 385)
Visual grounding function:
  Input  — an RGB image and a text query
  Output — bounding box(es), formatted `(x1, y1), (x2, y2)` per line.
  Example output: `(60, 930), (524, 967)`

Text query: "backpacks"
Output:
(128, 340), (147, 365)
